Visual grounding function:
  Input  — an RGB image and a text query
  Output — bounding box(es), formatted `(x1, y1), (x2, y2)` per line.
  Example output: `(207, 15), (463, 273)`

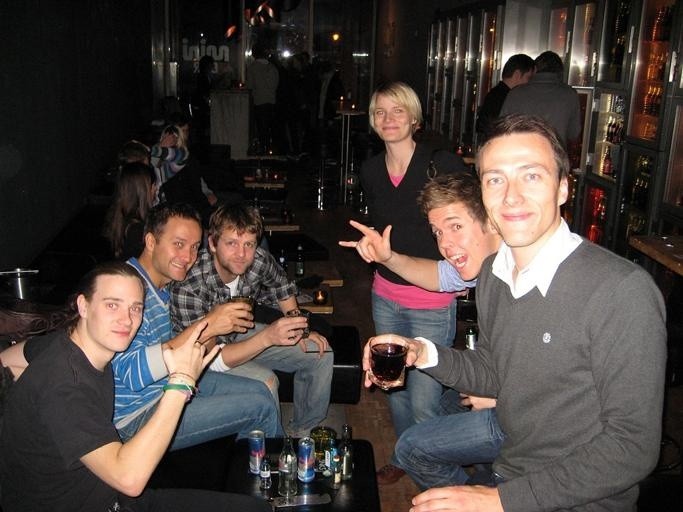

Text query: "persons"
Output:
(362, 113), (670, 512)
(167, 198), (336, 439)
(110, 200), (286, 452)
(500, 48), (580, 154)
(338, 170), (496, 415)
(354, 80), (466, 486)
(475, 52), (533, 141)
(106, 44), (349, 257)
(2, 261), (271, 512)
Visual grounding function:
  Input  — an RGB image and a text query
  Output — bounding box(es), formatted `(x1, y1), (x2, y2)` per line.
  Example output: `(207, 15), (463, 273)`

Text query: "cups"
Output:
(285, 308), (312, 340)
(369, 336), (408, 391)
(230, 296), (255, 323)
(308, 425), (338, 477)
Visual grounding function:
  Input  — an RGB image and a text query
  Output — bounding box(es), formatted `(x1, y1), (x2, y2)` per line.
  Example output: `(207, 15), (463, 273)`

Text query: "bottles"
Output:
(245, 195), (296, 222)
(562, 1), (676, 261)
(464, 326), (476, 353)
(277, 432), (299, 498)
(294, 242), (303, 277)
(259, 457), (272, 490)
(277, 249), (289, 273)
(327, 424), (354, 492)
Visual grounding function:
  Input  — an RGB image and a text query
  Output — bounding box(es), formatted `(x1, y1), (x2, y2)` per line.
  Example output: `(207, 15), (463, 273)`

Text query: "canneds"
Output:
(248, 430), (266, 475)
(297, 437), (316, 483)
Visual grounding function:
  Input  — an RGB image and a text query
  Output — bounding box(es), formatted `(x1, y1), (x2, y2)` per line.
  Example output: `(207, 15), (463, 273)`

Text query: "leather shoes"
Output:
(376, 464), (405, 485)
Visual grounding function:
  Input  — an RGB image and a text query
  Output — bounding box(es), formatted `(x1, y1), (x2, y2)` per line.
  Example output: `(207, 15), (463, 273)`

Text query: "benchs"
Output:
(1, 144), (232, 418)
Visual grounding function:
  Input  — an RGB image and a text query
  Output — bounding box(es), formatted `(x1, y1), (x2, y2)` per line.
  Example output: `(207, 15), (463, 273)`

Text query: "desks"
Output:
(224, 159), (380, 512)
(337, 110), (366, 207)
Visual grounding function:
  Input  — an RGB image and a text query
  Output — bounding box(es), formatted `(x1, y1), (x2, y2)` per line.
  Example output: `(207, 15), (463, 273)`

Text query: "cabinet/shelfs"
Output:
(425, 1), (683, 388)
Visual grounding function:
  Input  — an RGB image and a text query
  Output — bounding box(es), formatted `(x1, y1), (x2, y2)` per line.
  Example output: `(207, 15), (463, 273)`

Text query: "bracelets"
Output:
(161, 371), (197, 402)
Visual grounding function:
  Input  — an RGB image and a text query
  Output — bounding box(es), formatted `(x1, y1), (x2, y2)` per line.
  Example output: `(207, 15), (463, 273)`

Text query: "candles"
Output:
(352, 109), (355, 113)
(339, 101), (343, 111)
